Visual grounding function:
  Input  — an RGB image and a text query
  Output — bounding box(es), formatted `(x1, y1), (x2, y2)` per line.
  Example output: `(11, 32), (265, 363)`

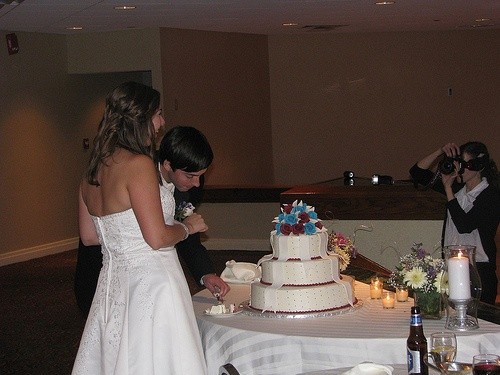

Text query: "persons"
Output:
(409, 141), (500, 304)
(74, 126), (231, 317)
(70, 81), (208, 375)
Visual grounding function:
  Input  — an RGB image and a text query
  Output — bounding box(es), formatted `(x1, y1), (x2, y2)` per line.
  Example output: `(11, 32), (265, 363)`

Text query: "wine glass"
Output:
(430, 332), (457, 375)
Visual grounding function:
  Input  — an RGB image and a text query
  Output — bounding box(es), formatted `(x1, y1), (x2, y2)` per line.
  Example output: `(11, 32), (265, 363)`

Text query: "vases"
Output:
(412, 288), (446, 320)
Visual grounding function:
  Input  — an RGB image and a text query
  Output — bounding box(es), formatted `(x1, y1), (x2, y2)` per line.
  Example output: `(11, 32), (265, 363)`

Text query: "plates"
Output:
(204, 308), (243, 318)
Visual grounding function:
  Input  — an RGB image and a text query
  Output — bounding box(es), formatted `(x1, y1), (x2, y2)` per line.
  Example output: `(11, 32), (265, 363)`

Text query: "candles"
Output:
(446, 249), (471, 299)
(397, 289), (408, 302)
(383, 294), (395, 308)
(370, 277), (383, 298)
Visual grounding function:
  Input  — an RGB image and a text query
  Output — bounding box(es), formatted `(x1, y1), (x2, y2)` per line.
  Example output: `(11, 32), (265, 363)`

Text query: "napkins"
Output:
(341, 360), (394, 375)
(220, 259), (263, 285)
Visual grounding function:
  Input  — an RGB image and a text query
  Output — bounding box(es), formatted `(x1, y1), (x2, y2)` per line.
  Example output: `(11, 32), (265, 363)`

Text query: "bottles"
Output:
(407, 306), (429, 375)
(440, 245), (482, 332)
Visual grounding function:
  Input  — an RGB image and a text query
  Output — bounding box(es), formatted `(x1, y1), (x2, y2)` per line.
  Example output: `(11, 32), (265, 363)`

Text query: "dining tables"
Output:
(192, 280), (500, 375)
(297, 362), (473, 375)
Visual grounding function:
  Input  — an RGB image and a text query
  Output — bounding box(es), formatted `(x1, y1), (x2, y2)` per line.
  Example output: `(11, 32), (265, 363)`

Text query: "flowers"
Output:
(327, 229), (356, 272)
(174, 200), (195, 222)
(385, 240), (450, 316)
(270, 199), (328, 236)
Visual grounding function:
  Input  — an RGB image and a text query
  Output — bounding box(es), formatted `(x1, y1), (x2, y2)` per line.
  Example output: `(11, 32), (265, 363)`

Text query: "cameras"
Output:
(438, 153), (465, 174)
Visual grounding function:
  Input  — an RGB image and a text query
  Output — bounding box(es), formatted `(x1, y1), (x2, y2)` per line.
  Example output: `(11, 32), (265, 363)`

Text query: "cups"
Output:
(396, 285), (408, 302)
(472, 354), (500, 375)
(371, 276), (383, 284)
(370, 284), (383, 299)
(381, 291), (395, 310)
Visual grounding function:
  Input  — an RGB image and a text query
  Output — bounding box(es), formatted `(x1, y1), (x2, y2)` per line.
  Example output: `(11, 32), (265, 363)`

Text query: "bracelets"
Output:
(176, 222), (189, 239)
